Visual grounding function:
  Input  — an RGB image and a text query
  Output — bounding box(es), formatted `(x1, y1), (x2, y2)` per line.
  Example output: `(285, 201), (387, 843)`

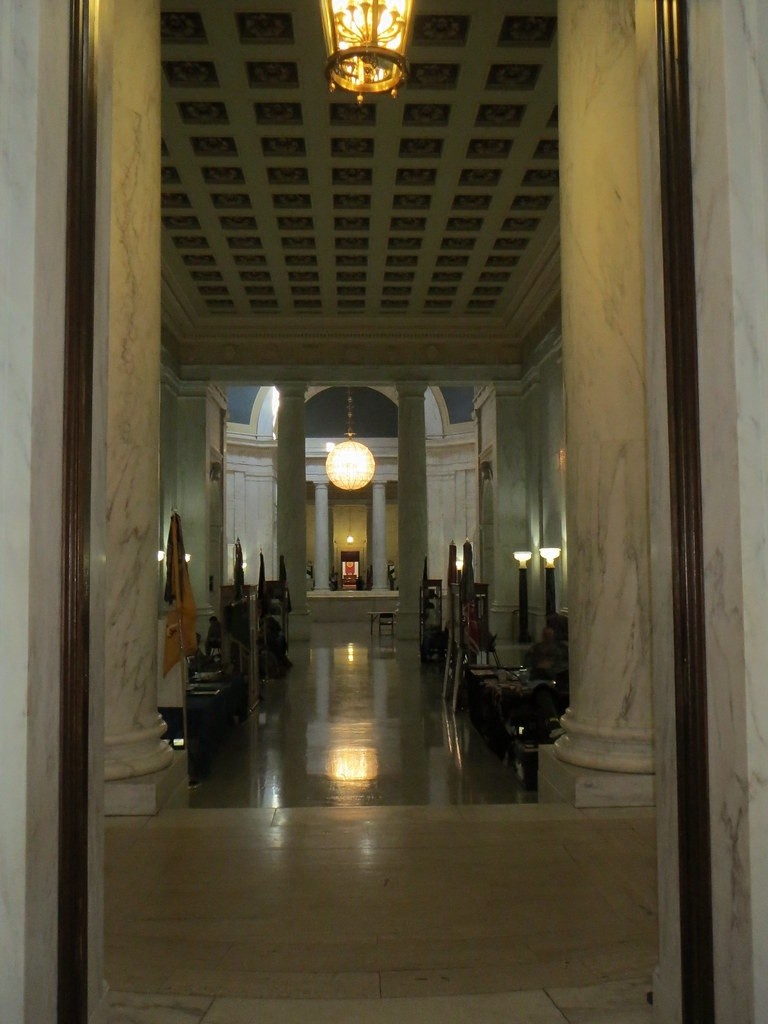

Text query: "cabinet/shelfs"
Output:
(449, 582), (488, 664)
(419, 579), (442, 650)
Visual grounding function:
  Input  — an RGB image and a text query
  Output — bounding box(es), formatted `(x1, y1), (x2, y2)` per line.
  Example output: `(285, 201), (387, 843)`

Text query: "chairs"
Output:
(379, 613), (394, 631)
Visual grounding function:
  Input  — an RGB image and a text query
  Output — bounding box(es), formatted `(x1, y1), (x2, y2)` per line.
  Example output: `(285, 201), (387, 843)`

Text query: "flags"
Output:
(163, 513), (198, 678)
(258, 553), (265, 599)
(448, 545), (457, 586)
(460, 542), (479, 654)
(234, 542), (244, 601)
(280, 554), (286, 595)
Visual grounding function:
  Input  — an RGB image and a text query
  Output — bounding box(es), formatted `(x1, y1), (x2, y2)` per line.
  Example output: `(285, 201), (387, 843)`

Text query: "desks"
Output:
(367, 612), (397, 635)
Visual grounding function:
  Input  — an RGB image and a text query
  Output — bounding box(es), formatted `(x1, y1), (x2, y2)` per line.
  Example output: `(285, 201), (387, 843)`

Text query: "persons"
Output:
(205, 616), (222, 656)
(388, 560), (396, 591)
(259, 608), (293, 669)
(525, 627), (563, 680)
(356, 576), (366, 591)
(533, 669), (570, 743)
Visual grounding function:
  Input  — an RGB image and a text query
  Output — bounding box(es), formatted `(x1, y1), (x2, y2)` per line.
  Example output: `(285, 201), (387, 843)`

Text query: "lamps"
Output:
(319, 1), (411, 106)
(326, 387), (376, 491)
(513, 551), (532, 569)
(347, 507), (354, 545)
(539, 547), (561, 568)
